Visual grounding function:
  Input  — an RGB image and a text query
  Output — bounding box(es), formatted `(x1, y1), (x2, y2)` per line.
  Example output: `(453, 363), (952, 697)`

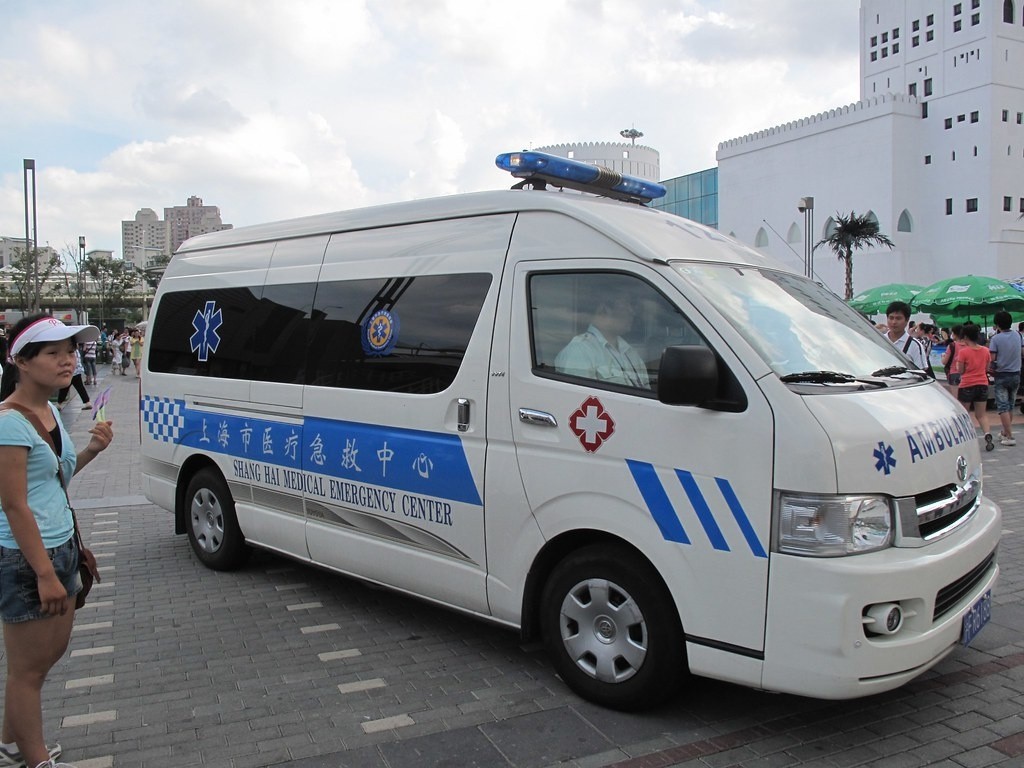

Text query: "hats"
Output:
(7, 317), (100, 365)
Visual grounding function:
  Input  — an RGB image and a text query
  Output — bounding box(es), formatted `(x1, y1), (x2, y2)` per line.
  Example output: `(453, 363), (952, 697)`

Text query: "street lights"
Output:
(797, 196), (815, 278)
(78, 235), (87, 326)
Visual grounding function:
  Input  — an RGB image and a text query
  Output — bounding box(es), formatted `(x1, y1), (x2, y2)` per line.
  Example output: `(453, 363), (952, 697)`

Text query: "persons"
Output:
(0, 319), (145, 411)
(0, 312), (113, 768)
(554, 274), (652, 391)
(867, 301), (1024, 451)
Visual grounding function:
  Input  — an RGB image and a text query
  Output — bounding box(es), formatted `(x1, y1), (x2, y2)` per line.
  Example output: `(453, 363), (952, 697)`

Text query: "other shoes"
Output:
(84, 380), (92, 385)
(984, 431), (995, 451)
(122, 373), (127, 376)
(1020, 401), (1024, 414)
(82, 402), (92, 410)
(94, 382), (97, 385)
(998, 430), (1007, 439)
(1001, 437), (1016, 446)
(136, 374), (139, 378)
(56, 405), (63, 411)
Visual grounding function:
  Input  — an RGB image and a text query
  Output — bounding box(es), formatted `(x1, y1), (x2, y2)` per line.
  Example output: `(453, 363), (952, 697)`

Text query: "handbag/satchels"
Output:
(81, 353), (86, 363)
(989, 360), (996, 377)
(122, 355), (130, 367)
(75, 549), (101, 610)
(119, 340), (125, 352)
(112, 357), (119, 370)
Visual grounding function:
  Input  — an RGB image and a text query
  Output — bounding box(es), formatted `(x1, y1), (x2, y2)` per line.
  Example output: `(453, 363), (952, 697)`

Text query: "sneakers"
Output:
(0, 743), (63, 768)
(27, 758), (76, 768)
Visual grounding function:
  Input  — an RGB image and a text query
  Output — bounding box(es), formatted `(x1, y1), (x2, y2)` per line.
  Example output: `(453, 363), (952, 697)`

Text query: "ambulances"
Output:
(136, 145), (1004, 713)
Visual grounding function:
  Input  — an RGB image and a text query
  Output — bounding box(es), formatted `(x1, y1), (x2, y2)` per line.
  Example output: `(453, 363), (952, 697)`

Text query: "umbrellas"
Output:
(844, 274), (1024, 345)
